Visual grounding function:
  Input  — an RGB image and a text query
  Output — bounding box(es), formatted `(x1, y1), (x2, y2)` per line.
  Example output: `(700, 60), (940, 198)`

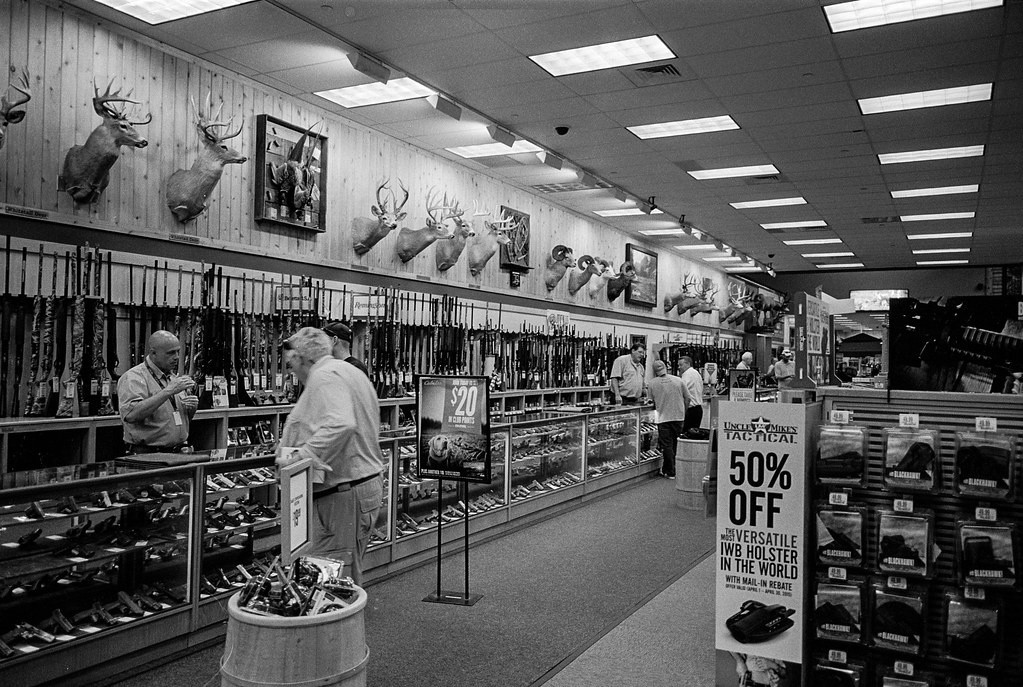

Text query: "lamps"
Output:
(576, 170), (596, 189)
(740, 254), (750, 264)
(486, 124), (516, 147)
(680, 225), (692, 236)
(713, 240), (723, 252)
(346, 50), (391, 85)
(725, 247), (736, 257)
(426, 92), (461, 121)
(636, 201), (651, 215)
(693, 232), (707, 242)
(767, 270), (776, 277)
(759, 265), (767, 272)
(607, 187), (627, 202)
(535, 150), (563, 170)
(749, 259), (759, 268)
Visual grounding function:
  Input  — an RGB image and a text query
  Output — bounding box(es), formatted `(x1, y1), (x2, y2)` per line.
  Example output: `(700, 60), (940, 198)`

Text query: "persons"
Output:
(766, 349), (795, 391)
(273, 326), (384, 588)
(609, 342), (648, 405)
(736, 351), (753, 370)
(116, 329), (199, 456)
(677, 356), (704, 432)
(835, 361), (882, 377)
(320, 322), (369, 378)
(647, 360), (691, 480)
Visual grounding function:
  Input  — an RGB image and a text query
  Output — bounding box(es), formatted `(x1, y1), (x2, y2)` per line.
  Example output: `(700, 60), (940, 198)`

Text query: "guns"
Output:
(1, 228), (754, 656)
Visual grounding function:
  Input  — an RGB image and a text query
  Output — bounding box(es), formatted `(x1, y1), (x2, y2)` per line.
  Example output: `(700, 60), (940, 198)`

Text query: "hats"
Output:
(653, 361), (666, 376)
(322, 322), (351, 343)
(782, 350), (791, 356)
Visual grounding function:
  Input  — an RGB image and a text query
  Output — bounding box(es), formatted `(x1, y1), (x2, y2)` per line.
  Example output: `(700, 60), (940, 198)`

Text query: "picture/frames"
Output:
(625, 243), (658, 307)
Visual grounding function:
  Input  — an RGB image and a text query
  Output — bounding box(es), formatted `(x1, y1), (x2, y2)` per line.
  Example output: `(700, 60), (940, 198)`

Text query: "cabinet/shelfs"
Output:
(0, 387), (779, 687)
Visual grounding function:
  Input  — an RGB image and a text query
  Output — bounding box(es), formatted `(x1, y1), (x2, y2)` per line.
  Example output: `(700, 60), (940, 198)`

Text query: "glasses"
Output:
(283, 340), (295, 350)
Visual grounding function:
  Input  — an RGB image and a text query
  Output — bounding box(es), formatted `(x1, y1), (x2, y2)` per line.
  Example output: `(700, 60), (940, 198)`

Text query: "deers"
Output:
(165, 91), (248, 224)
(0, 64), (153, 207)
(664, 272), (763, 326)
(351, 176), (521, 277)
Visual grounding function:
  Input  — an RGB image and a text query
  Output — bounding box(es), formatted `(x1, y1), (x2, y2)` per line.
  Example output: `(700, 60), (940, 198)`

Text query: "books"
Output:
(115, 451), (210, 469)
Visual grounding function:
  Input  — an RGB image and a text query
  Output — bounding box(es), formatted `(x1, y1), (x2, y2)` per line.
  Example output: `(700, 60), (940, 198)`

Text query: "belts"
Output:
(313, 473), (378, 499)
(132, 445), (181, 453)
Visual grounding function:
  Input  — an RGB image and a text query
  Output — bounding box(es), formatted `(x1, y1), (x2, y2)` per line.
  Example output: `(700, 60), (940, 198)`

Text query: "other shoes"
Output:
(660, 467), (676, 479)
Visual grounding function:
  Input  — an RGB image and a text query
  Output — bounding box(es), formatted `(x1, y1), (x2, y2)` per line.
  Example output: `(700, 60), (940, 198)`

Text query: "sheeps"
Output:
(544, 245), (640, 302)
